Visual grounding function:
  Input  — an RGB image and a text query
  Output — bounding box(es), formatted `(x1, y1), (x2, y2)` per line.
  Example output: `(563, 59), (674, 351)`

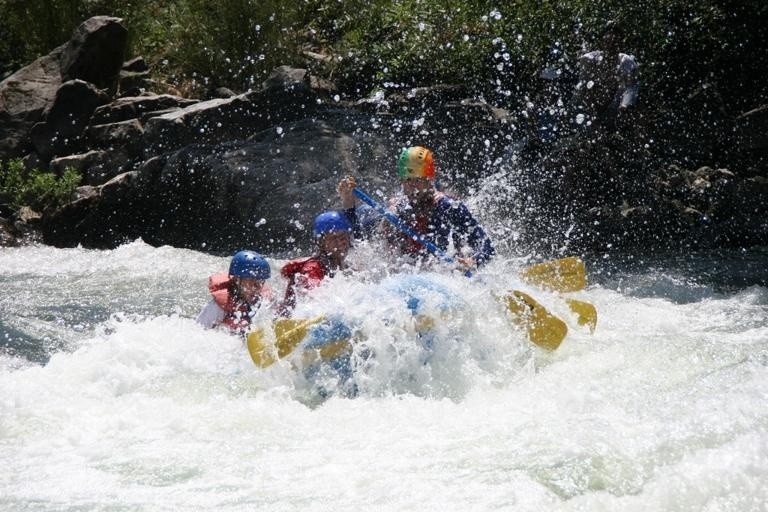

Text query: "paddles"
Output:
(519, 257), (585, 294)
(246, 316), (325, 368)
(354, 190), (567, 352)
(564, 297), (597, 333)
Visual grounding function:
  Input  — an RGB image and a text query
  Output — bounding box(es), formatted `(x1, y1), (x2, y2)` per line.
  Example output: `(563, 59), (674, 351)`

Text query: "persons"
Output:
(195, 248), (301, 341)
(278, 210), (365, 327)
(336, 148), (496, 281)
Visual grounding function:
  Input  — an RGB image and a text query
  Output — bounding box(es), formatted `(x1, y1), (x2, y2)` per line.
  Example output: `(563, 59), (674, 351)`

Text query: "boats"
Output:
(304, 275), (461, 405)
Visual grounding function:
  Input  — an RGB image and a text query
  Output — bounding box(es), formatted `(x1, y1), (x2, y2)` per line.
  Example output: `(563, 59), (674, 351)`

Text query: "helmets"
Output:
(314, 211), (354, 236)
(396, 146), (436, 180)
(230, 250), (271, 279)
